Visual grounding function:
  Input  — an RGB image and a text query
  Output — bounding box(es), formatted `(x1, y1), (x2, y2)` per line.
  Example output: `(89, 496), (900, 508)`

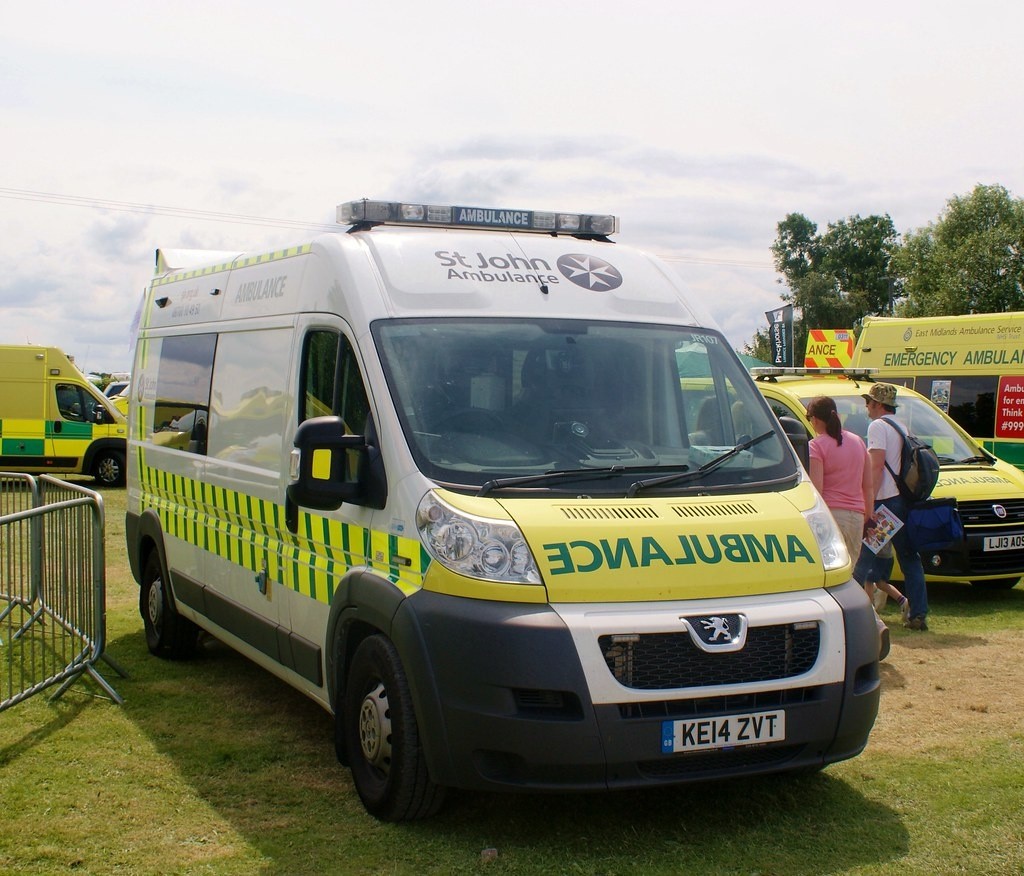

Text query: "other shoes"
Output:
(876, 619), (890, 661)
(903, 614), (928, 631)
(900, 598), (909, 621)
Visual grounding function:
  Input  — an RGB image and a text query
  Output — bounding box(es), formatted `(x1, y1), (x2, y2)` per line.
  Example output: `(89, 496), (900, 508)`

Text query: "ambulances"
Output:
(116, 196), (892, 824)
(795, 314), (1024, 479)
(1, 343), (151, 489)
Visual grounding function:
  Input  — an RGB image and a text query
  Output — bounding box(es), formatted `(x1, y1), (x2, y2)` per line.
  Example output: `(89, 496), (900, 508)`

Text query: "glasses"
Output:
(866, 398), (879, 405)
(806, 412), (821, 421)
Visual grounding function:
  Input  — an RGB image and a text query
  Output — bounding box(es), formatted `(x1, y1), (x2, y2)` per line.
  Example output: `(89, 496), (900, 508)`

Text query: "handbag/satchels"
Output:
(906, 498), (963, 552)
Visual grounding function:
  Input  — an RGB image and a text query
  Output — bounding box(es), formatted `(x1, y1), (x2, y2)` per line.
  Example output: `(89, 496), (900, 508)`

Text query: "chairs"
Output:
(513, 340), (618, 446)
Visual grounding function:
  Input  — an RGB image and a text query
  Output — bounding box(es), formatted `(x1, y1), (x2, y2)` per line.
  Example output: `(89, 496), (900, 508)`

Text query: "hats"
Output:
(861, 382), (899, 407)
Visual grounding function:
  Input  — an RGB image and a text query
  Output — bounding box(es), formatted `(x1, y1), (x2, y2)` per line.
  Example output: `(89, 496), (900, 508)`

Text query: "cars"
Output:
(85, 372), (133, 420)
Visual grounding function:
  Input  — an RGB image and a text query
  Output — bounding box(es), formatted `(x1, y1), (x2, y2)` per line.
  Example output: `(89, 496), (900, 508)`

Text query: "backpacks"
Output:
(875, 417), (940, 505)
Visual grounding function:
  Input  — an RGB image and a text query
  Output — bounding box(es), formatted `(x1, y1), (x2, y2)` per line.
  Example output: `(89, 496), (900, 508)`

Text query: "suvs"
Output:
(670, 366), (1024, 599)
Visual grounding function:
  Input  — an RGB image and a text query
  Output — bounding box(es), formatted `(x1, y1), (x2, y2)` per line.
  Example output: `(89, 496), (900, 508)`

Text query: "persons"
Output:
(689, 394), (756, 451)
(807, 382), (966, 661)
